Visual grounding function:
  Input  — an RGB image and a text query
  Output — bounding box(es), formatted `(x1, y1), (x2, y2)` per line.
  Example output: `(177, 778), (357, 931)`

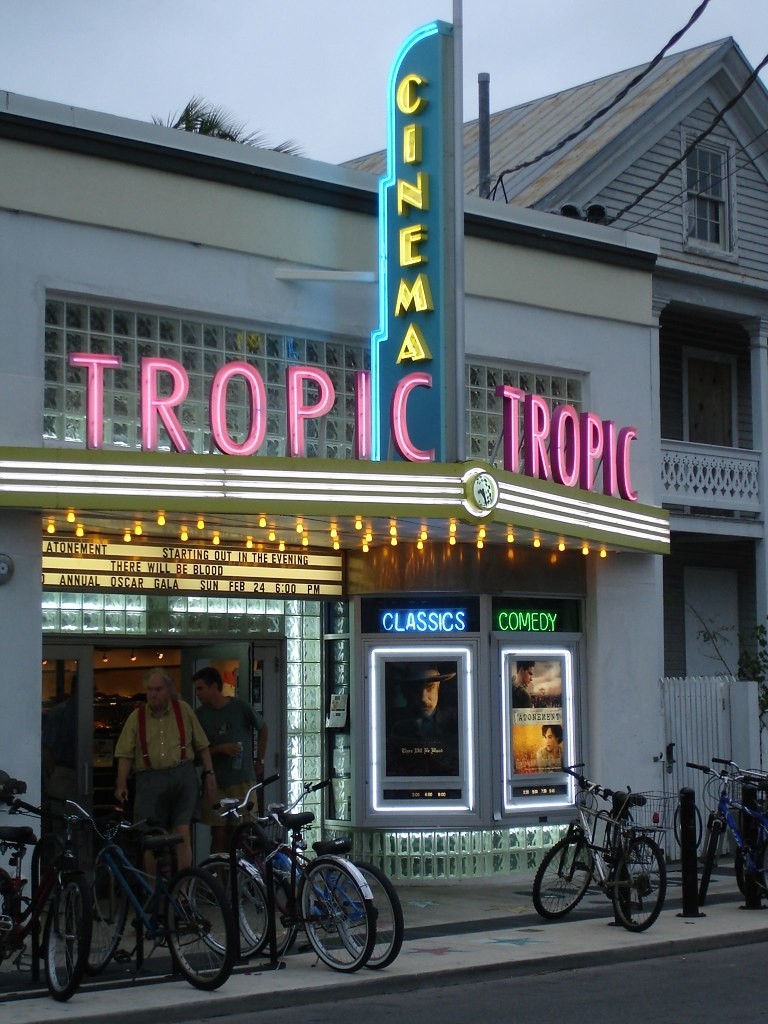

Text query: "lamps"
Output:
(42, 646), (165, 666)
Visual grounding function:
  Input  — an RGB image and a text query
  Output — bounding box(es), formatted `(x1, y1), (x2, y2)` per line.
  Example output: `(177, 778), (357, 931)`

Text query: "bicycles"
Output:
(685, 757), (768, 905)
(531, 763), (689, 933)
(0, 768), (405, 1003)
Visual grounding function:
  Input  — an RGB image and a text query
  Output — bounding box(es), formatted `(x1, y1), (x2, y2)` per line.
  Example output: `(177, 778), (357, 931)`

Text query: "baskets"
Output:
(731, 768), (768, 804)
(628, 790), (681, 830)
(235, 809), (289, 856)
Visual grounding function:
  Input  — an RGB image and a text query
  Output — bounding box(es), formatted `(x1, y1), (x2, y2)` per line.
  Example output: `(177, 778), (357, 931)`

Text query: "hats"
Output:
(392, 662), (457, 687)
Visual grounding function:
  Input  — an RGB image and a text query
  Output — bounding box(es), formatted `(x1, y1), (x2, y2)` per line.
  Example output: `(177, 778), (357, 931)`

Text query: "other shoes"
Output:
(143, 893), (154, 913)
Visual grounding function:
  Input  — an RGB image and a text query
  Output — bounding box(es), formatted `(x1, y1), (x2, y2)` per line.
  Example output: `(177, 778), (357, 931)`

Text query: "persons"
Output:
(132, 693), (147, 710)
(191, 667), (268, 901)
(535, 723), (564, 772)
(41, 673), (99, 913)
(512, 659), (535, 707)
(114, 667), (217, 915)
(387, 663), (459, 779)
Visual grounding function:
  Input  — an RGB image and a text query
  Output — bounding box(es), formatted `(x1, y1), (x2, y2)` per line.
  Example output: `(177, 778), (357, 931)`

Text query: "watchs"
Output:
(204, 770), (215, 774)
(256, 758), (265, 764)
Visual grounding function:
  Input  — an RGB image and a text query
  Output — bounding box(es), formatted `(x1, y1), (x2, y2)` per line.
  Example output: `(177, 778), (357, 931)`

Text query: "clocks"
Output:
(461, 471), (499, 517)
